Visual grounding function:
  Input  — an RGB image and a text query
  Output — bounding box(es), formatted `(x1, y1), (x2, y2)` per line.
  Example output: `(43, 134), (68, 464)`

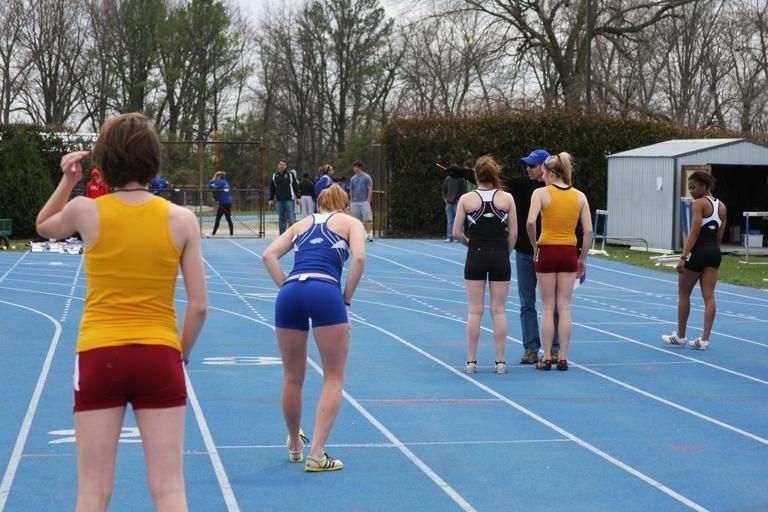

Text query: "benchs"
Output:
(0, 218), (13, 250)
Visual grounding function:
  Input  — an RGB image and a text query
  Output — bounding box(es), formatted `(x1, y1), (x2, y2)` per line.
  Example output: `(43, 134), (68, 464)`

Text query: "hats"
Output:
(521, 149), (550, 165)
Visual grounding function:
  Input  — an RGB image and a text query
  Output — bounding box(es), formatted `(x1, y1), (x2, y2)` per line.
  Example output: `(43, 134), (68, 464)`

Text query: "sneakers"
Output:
(495, 361), (507, 374)
(661, 333), (687, 345)
(304, 452), (343, 471)
(689, 337), (710, 349)
(520, 350), (569, 369)
(466, 360), (479, 373)
(287, 427), (308, 462)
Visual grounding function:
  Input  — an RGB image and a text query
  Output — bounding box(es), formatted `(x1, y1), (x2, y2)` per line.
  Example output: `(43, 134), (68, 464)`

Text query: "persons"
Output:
(445, 148), (561, 365)
(267, 160), (374, 243)
(207, 167), (235, 238)
(453, 153), (519, 376)
(28, 114), (207, 512)
(145, 170), (170, 196)
(440, 160), (469, 243)
(659, 169), (728, 352)
(82, 167), (112, 200)
(259, 181), (366, 472)
(464, 158), (478, 194)
(525, 150), (594, 372)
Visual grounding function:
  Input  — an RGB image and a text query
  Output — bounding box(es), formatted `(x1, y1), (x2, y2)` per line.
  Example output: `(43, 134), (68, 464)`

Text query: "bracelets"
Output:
(344, 301), (351, 307)
(680, 255), (686, 260)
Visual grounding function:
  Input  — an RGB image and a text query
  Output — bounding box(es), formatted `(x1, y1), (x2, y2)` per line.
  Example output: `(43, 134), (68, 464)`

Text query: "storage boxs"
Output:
(742, 234), (763, 248)
